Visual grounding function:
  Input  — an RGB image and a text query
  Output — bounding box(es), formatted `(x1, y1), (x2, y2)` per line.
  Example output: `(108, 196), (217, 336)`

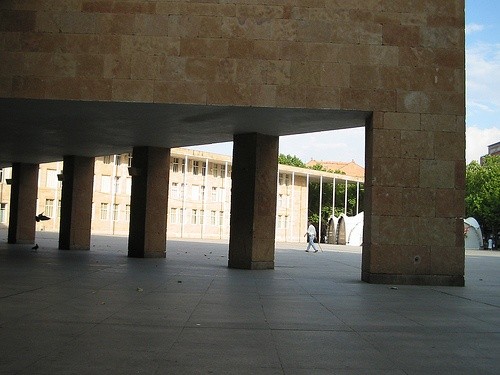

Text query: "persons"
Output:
(303, 220), (319, 253)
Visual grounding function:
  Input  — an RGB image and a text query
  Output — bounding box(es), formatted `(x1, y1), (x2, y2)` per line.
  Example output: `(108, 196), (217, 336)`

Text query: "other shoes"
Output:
(305, 250), (309, 252)
(314, 250), (318, 253)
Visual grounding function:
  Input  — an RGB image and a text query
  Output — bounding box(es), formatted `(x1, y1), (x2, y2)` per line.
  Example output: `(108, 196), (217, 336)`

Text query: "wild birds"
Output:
(39, 214), (50, 220)
(32, 244), (39, 249)
(35, 212), (44, 222)
